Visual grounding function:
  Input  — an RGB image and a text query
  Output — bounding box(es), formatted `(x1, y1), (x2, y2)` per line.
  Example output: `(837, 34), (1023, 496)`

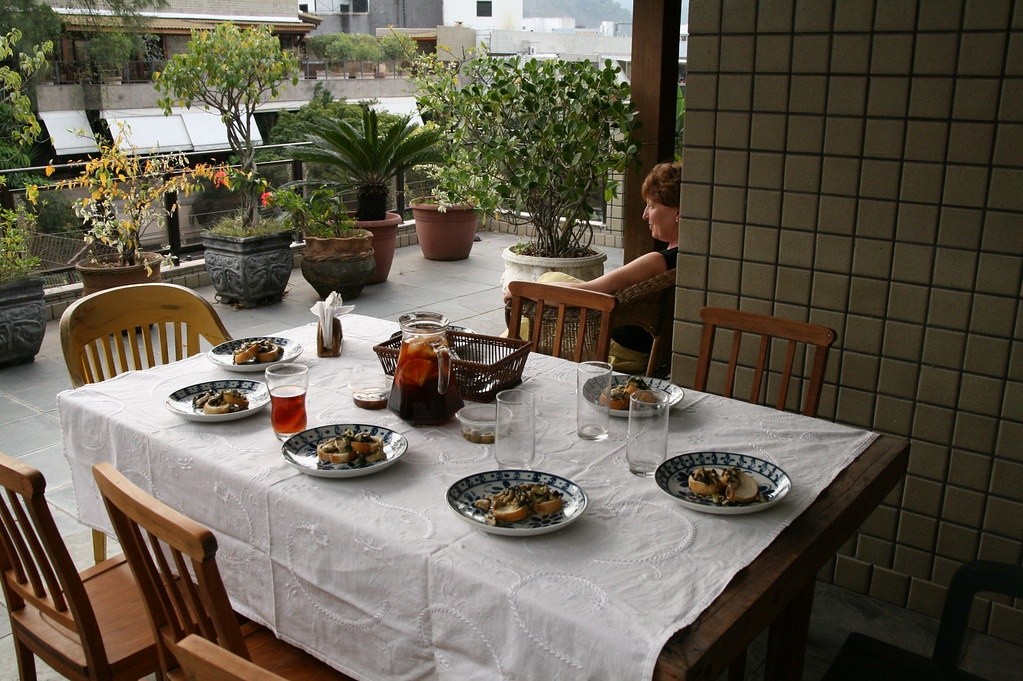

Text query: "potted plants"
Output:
(280, 185), (374, 302)
(149, 19), (302, 312)
(23, 116), (269, 337)
(0, 27), (54, 369)
(416, 53), (641, 297)
(276, 103), (452, 285)
(385, 23), (493, 262)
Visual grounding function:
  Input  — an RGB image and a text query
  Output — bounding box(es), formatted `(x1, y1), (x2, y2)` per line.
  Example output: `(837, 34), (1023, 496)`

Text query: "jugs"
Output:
(387, 311), (464, 429)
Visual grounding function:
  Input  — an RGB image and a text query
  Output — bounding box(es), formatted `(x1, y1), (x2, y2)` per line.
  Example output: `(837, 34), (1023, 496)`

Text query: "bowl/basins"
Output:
(347, 375), (393, 409)
(455, 404), (514, 444)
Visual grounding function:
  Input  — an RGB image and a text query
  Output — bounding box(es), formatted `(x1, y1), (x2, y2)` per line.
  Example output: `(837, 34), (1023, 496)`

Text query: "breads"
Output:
(600, 385), (657, 408)
(318, 435), (387, 463)
(492, 493), (562, 522)
(236, 340), (280, 363)
(196, 392), (250, 414)
(688, 469), (759, 502)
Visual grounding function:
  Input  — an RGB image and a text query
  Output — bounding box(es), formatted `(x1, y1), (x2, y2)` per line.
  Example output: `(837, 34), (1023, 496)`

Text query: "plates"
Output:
(280, 423), (408, 478)
(163, 378), (272, 422)
(388, 324), (477, 350)
(445, 470), (588, 537)
(208, 336), (303, 372)
(581, 375), (685, 418)
(654, 452), (792, 515)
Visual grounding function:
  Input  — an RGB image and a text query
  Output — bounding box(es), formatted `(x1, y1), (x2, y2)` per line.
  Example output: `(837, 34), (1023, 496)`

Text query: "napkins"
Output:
(309, 291), (356, 350)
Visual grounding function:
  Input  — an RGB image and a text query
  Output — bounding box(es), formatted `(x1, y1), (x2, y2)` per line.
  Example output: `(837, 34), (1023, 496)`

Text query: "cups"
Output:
(265, 363), (309, 442)
(626, 389), (670, 478)
(576, 362), (613, 441)
(495, 390), (536, 469)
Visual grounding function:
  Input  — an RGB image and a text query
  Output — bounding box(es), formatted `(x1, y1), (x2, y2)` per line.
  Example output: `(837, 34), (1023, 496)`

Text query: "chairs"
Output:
(506, 281), (617, 365)
(58, 282), (234, 567)
(823, 554), (1023, 681)
(1, 448), (348, 680)
(505, 267), (677, 379)
(692, 306), (837, 418)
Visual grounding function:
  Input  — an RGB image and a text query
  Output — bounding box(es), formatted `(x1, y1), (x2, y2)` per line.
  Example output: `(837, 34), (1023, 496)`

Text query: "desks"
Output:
(59, 306), (911, 681)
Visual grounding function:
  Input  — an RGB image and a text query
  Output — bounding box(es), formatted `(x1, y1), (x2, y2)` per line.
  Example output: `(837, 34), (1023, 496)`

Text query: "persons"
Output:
(498, 159), (683, 370)
